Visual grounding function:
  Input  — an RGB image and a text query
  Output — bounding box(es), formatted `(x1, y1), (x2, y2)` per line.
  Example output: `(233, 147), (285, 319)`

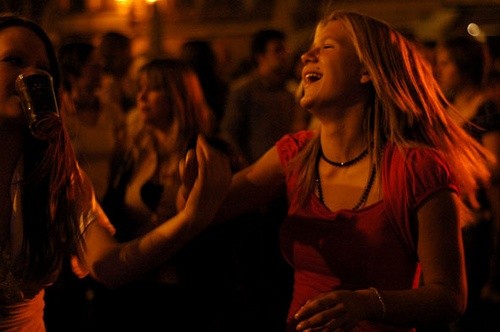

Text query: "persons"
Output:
(0, 0), (500, 332)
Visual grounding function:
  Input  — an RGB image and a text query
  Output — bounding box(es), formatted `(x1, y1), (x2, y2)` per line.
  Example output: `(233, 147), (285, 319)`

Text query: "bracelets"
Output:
(370, 287), (390, 322)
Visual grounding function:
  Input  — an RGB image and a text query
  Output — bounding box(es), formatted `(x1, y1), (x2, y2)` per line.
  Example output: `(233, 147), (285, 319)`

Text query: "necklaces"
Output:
(315, 149), (376, 210)
(318, 141), (375, 168)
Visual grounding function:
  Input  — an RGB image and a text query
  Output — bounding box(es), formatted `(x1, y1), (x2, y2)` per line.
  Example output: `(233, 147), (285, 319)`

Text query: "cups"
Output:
(16, 71), (61, 137)
